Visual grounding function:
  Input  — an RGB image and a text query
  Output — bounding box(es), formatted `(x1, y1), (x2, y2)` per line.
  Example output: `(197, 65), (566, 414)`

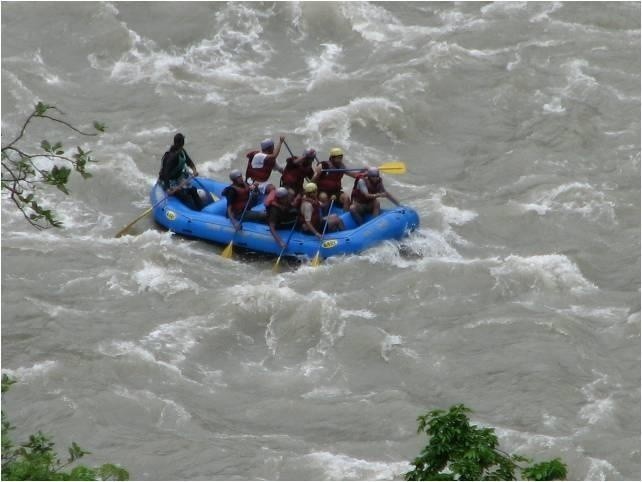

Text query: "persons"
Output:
(161, 133), (204, 210)
(221, 135), (408, 248)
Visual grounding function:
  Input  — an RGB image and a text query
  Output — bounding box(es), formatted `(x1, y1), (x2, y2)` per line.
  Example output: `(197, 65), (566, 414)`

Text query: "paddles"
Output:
(115, 175), (195, 238)
(312, 199), (334, 266)
(273, 216), (299, 272)
(187, 171), (219, 203)
(320, 162), (406, 174)
(221, 190), (253, 258)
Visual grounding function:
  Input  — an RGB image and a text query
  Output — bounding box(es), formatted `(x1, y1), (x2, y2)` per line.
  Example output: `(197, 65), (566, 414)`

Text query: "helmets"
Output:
(229, 138), (380, 198)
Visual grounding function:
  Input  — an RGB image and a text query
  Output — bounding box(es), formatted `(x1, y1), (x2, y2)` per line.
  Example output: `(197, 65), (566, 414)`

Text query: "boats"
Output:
(149, 175), (420, 267)
